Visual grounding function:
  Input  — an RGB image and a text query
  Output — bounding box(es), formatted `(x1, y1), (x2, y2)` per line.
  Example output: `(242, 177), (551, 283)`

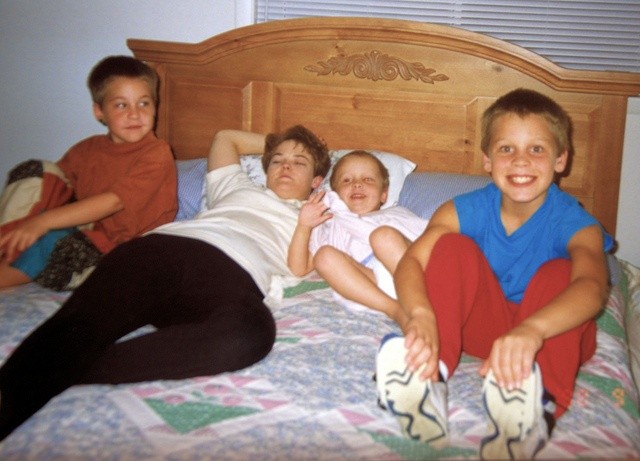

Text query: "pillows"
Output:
(238, 149), (418, 212)
(174, 159), (208, 222)
(398, 172), (495, 221)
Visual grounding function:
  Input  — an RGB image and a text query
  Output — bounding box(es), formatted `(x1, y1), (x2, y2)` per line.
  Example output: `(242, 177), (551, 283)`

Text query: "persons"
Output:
(0, 54), (180, 294)
(373, 88), (612, 460)
(287, 150), (430, 329)
(1, 122), (332, 452)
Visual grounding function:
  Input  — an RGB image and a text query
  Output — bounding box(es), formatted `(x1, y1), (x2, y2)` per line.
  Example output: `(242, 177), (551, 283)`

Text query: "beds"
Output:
(0, 17), (639, 461)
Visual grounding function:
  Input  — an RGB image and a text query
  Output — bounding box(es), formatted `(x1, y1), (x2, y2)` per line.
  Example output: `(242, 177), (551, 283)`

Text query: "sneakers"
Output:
(479, 362), (544, 460)
(375, 333), (448, 451)
